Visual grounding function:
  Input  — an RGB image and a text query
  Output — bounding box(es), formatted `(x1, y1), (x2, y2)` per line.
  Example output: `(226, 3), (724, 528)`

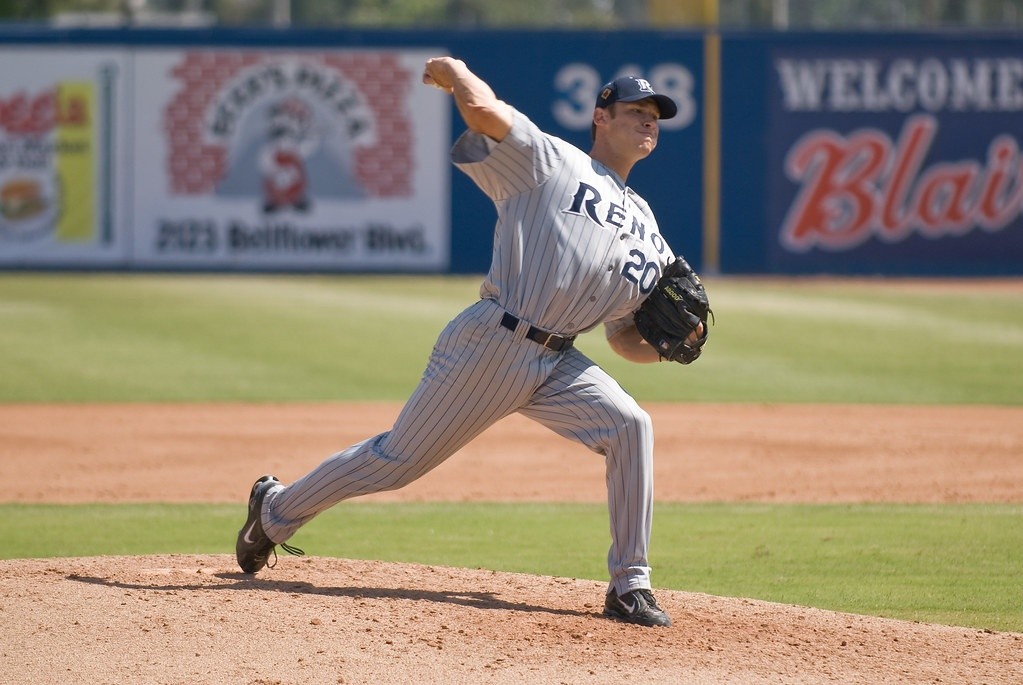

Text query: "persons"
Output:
(236, 56), (709, 628)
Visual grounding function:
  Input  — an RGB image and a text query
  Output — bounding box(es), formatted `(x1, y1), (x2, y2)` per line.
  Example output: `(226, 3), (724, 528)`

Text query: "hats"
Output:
(595, 76), (677, 120)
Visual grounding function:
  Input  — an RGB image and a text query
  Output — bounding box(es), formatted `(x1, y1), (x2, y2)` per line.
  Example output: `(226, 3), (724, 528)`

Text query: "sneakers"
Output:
(236, 476), (305, 573)
(602, 587), (672, 627)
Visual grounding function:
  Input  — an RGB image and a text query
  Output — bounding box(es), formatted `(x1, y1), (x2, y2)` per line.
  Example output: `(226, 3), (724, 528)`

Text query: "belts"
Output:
(501, 312), (575, 352)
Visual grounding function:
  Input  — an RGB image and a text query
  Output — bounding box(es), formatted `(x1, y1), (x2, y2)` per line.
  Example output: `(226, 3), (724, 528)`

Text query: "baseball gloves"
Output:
(634, 254), (710, 364)
